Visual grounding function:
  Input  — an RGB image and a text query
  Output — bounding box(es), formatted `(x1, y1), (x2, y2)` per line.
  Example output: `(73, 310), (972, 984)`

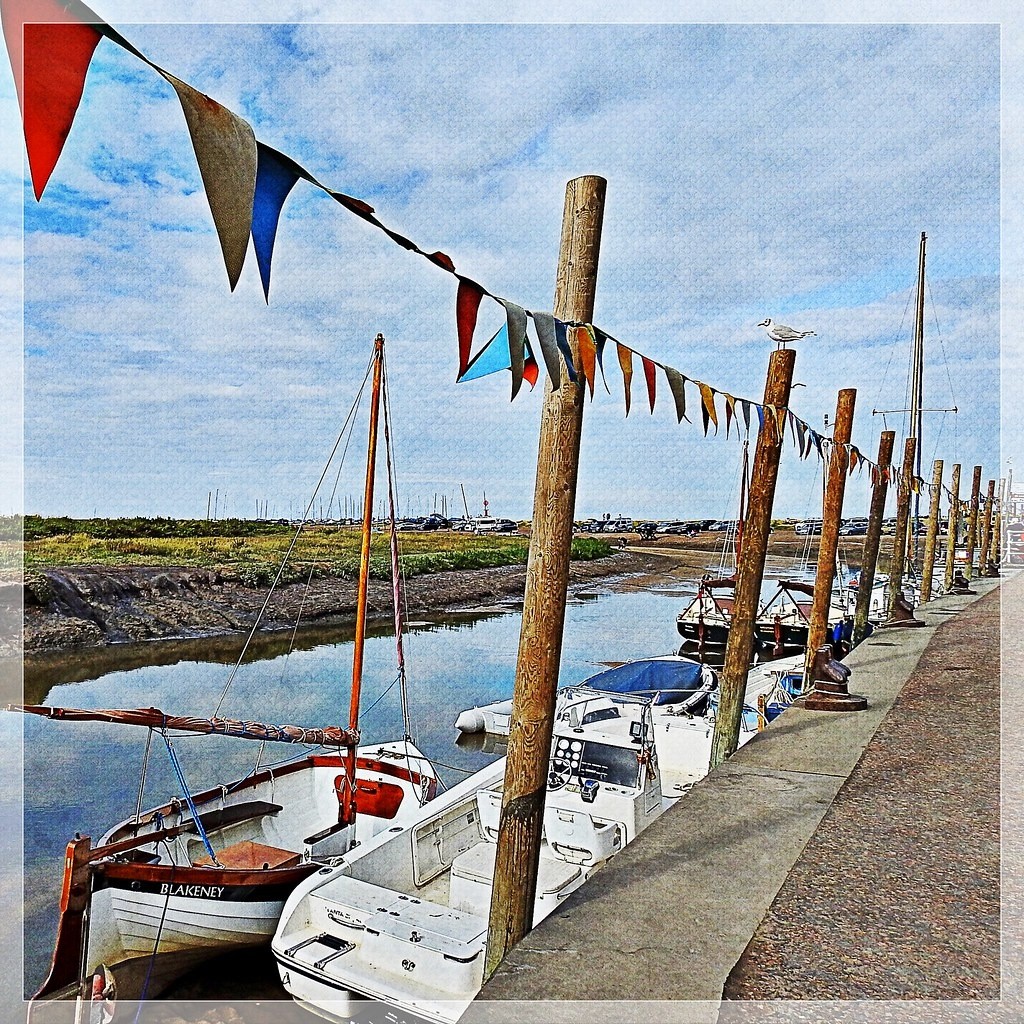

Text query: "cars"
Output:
(788, 518), (950, 535)
(572, 518), (744, 535)
(395, 514), (517, 532)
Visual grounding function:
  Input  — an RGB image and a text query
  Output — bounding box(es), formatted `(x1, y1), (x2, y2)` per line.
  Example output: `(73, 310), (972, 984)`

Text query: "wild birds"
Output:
(757, 318), (818, 353)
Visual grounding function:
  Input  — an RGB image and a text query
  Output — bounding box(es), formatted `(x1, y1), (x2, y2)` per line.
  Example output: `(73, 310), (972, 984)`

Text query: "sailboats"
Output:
(675, 425), (989, 650)
(24, 328), (438, 1023)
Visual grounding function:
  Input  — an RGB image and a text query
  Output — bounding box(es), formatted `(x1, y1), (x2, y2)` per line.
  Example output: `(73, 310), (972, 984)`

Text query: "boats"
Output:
(454, 654), (715, 739)
(270, 694), (769, 1023)
(708, 653), (807, 725)
